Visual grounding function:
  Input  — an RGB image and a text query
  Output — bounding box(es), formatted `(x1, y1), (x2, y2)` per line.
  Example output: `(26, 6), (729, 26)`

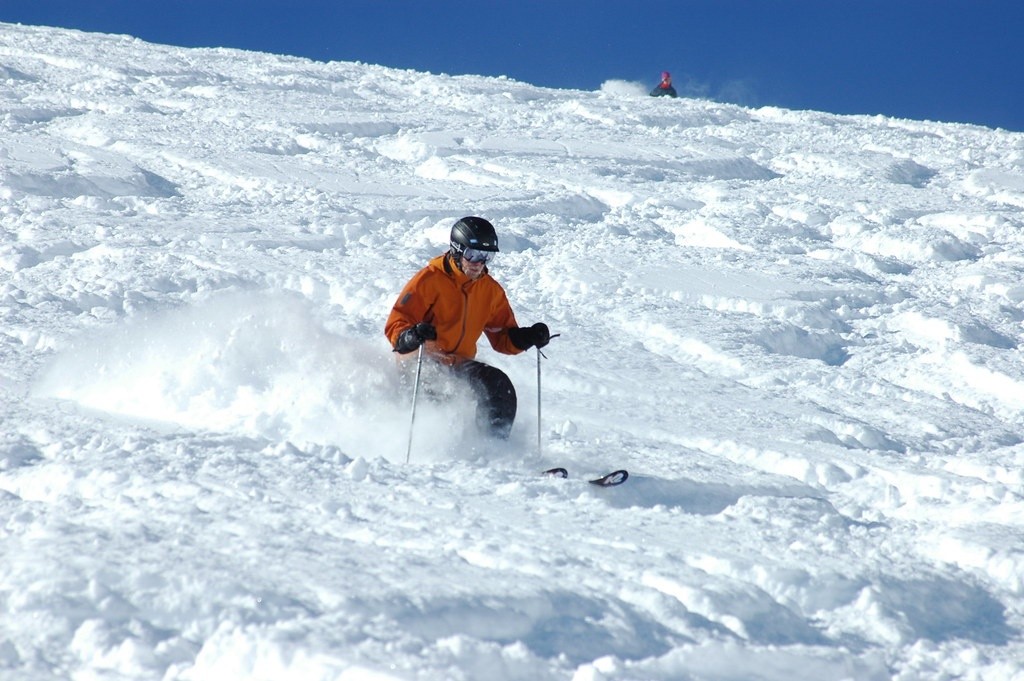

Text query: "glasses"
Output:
(461, 247), (495, 265)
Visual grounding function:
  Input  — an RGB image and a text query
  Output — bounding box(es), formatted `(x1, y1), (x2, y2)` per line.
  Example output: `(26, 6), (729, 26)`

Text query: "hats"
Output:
(662, 72), (670, 79)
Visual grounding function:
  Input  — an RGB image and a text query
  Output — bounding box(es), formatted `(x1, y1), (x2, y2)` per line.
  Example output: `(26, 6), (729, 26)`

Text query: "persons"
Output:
(386, 216), (550, 440)
(650, 72), (677, 98)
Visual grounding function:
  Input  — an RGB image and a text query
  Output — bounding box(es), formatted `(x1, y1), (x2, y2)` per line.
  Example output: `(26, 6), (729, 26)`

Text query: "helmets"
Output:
(450, 216), (500, 263)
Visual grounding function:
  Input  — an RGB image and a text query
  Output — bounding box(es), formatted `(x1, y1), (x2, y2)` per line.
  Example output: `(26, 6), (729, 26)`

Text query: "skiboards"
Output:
(541, 467), (630, 488)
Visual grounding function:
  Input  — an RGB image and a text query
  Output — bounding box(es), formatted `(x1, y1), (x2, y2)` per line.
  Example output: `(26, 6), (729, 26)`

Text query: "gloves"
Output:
(508, 322), (549, 351)
(396, 322), (437, 354)
